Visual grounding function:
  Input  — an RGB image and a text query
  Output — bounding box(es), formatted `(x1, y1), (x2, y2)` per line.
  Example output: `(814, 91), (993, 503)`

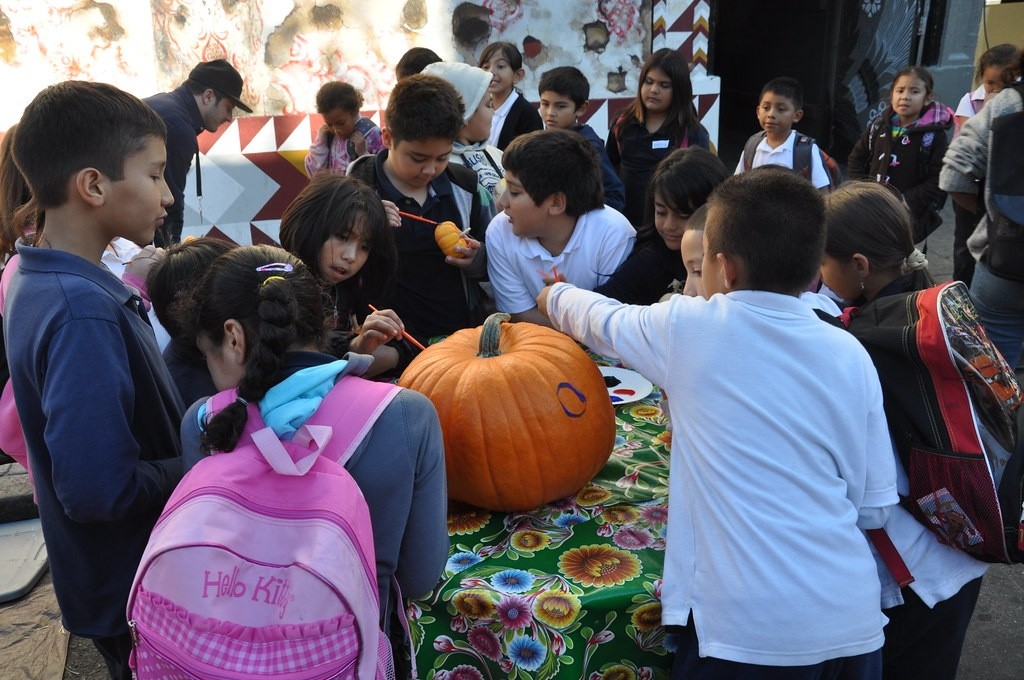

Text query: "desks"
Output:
(382, 345), (680, 680)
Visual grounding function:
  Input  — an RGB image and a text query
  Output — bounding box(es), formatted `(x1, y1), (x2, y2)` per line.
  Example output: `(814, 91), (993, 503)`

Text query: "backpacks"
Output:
(133, 377), (404, 680)
(745, 130), (843, 190)
(983, 82), (1024, 282)
(814, 279), (1024, 566)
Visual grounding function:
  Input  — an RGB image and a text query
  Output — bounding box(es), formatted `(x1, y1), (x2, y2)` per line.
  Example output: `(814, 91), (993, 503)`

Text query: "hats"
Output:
(190, 59), (253, 113)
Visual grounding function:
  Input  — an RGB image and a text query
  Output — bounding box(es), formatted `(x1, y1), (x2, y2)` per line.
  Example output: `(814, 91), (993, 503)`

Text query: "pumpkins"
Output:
(395, 312), (615, 513)
(434, 220), (471, 258)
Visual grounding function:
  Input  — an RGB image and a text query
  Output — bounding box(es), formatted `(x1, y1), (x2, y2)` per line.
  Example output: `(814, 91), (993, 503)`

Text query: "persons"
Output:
(733, 78), (831, 193)
(303, 41), (626, 300)
(847, 67), (956, 258)
(146, 236), (245, 409)
(938, 44), (1024, 372)
(324, 77), (487, 345)
(534, 168), (901, 680)
(676, 198), (991, 680)
(1, 79), (198, 678)
(179, 245), (451, 680)
(484, 126), (639, 351)
(140, 58), (255, 249)
(594, 47), (726, 308)
(279, 169), (407, 376)
(819, 181), (990, 302)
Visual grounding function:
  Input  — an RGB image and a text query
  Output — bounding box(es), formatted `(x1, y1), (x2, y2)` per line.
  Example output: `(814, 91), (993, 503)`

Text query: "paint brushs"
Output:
(369, 304), (426, 349)
(396, 211), (439, 226)
